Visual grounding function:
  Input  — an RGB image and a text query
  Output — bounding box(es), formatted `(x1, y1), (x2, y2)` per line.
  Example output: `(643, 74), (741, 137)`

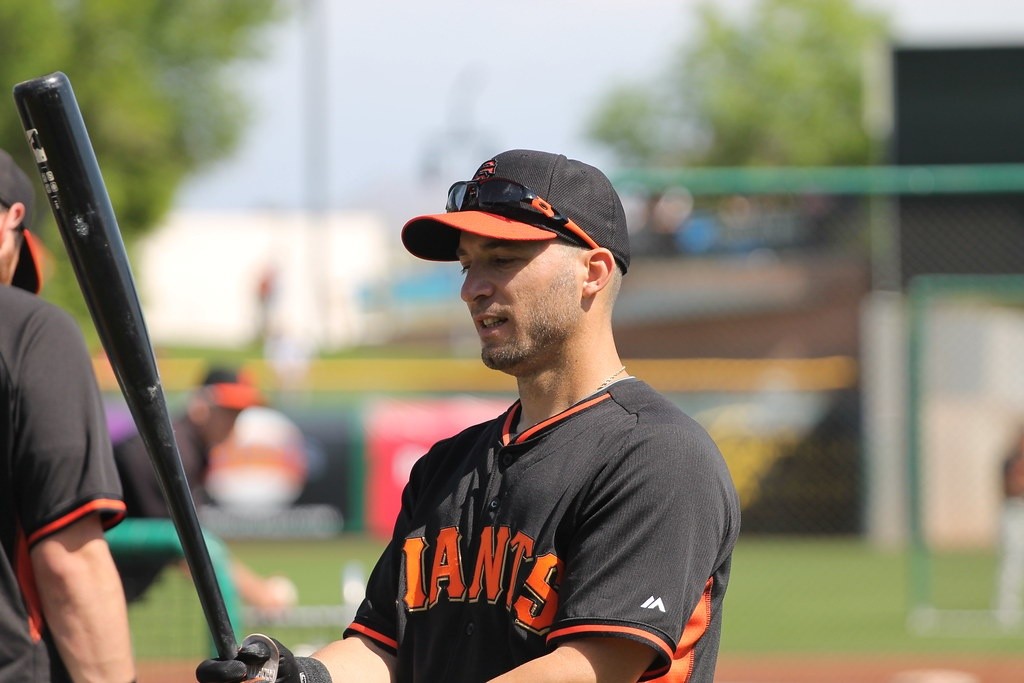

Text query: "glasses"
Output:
(445, 177), (600, 250)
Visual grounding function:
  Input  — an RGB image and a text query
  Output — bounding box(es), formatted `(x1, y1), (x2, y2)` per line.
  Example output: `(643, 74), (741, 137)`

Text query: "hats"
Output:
(1, 151), (42, 293)
(401, 150), (632, 274)
(202, 365), (260, 406)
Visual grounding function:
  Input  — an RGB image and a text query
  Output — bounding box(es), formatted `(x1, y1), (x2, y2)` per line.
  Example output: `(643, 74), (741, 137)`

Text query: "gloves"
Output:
(196, 633), (332, 683)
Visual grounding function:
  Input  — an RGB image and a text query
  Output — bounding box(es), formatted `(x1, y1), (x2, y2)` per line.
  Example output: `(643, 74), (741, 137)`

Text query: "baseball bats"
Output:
(10, 69), (239, 661)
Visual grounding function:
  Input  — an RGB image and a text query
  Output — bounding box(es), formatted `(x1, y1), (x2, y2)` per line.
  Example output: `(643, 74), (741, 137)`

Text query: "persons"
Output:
(103, 366), (261, 602)
(195, 147), (741, 683)
(0, 149), (134, 683)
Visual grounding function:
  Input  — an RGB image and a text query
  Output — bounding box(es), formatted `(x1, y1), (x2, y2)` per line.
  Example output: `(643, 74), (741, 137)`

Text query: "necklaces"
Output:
(595, 365), (626, 392)
